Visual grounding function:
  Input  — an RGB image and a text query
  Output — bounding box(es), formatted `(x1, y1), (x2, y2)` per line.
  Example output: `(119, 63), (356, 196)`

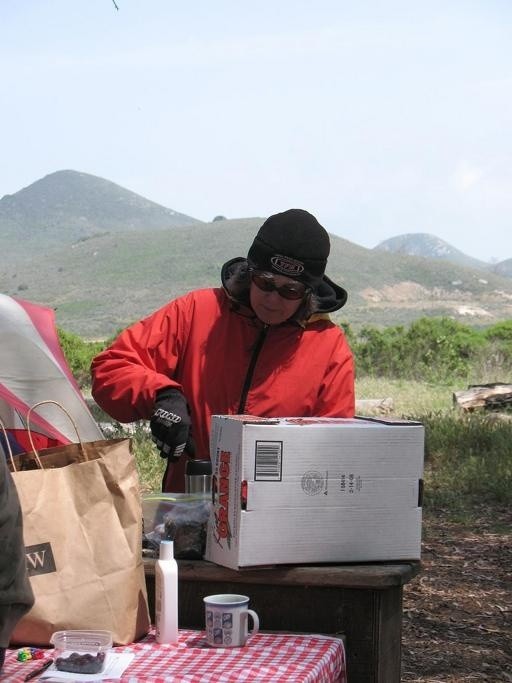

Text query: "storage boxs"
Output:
(204, 412), (426, 571)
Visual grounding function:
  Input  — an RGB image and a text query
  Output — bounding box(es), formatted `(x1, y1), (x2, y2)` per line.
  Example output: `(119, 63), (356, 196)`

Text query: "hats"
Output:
(220, 208), (348, 315)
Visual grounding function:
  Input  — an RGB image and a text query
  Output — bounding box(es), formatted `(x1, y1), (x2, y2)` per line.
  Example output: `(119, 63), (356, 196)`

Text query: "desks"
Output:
(1, 627), (344, 683)
(138, 561), (422, 682)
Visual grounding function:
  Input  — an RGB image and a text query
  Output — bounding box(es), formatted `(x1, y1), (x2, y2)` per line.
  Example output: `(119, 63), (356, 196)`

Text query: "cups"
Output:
(202, 593), (260, 649)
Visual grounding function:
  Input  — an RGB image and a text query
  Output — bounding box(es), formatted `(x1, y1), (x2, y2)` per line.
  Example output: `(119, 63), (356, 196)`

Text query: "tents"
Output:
(1, 291), (104, 449)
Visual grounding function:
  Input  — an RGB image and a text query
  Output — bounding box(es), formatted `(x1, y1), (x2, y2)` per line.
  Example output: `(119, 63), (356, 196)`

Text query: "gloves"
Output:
(149, 385), (190, 461)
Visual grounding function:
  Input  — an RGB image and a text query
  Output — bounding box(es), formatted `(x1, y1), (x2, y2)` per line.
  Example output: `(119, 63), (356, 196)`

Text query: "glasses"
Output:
(253, 268), (306, 302)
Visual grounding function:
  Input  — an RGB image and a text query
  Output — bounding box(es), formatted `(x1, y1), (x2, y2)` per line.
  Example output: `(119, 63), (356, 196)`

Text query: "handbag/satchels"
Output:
(0, 398), (154, 649)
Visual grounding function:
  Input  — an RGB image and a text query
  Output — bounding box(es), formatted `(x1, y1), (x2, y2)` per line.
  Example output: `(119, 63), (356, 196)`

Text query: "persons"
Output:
(90, 207), (358, 531)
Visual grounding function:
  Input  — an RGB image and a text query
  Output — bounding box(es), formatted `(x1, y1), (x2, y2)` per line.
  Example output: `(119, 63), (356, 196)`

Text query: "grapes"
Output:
(56, 652), (105, 674)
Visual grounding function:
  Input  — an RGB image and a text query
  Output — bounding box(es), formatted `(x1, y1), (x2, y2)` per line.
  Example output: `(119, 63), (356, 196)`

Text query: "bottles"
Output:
(184, 458), (212, 495)
(153, 537), (182, 645)
